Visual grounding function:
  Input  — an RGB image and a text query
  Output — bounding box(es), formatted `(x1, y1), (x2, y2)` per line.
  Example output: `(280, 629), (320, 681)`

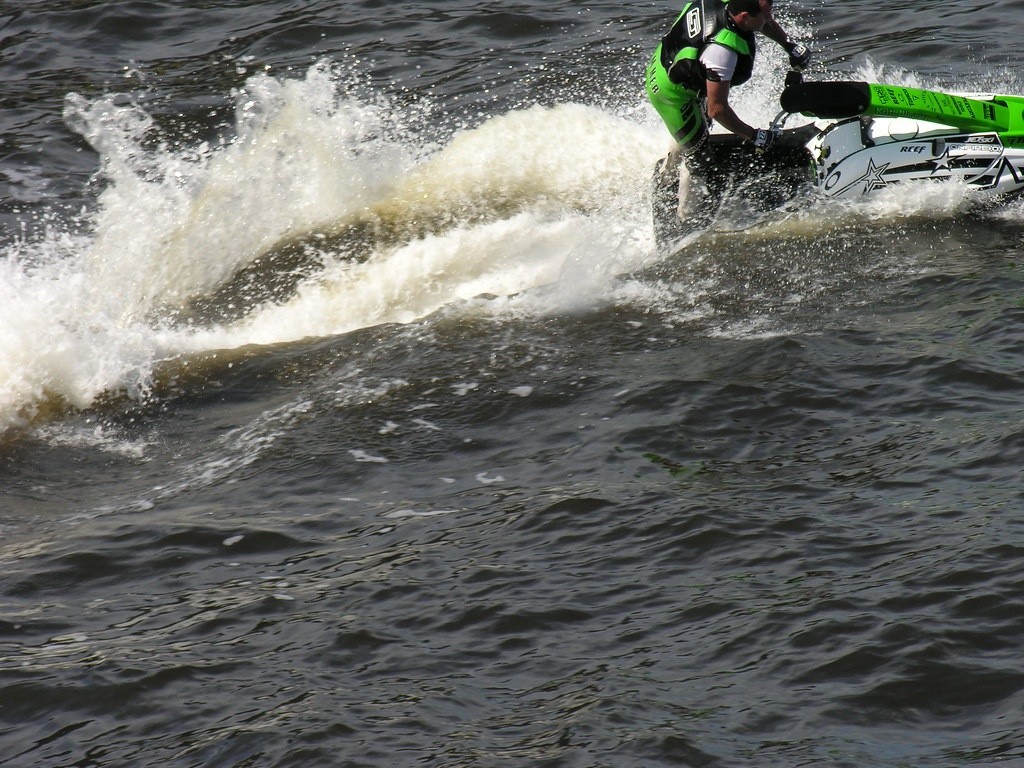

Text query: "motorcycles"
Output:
(650, 57), (1023, 256)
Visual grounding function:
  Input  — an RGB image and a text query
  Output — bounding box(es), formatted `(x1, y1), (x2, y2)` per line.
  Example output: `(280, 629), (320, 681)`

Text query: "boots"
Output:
(659, 139), (683, 185)
(677, 161), (707, 220)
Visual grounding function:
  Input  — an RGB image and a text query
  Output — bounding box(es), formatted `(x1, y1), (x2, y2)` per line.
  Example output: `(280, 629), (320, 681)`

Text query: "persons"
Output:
(645, 0), (812, 219)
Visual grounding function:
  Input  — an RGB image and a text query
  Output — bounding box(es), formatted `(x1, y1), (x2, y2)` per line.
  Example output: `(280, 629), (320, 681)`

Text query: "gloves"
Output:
(785, 43), (812, 70)
(750, 129), (779, 152)
